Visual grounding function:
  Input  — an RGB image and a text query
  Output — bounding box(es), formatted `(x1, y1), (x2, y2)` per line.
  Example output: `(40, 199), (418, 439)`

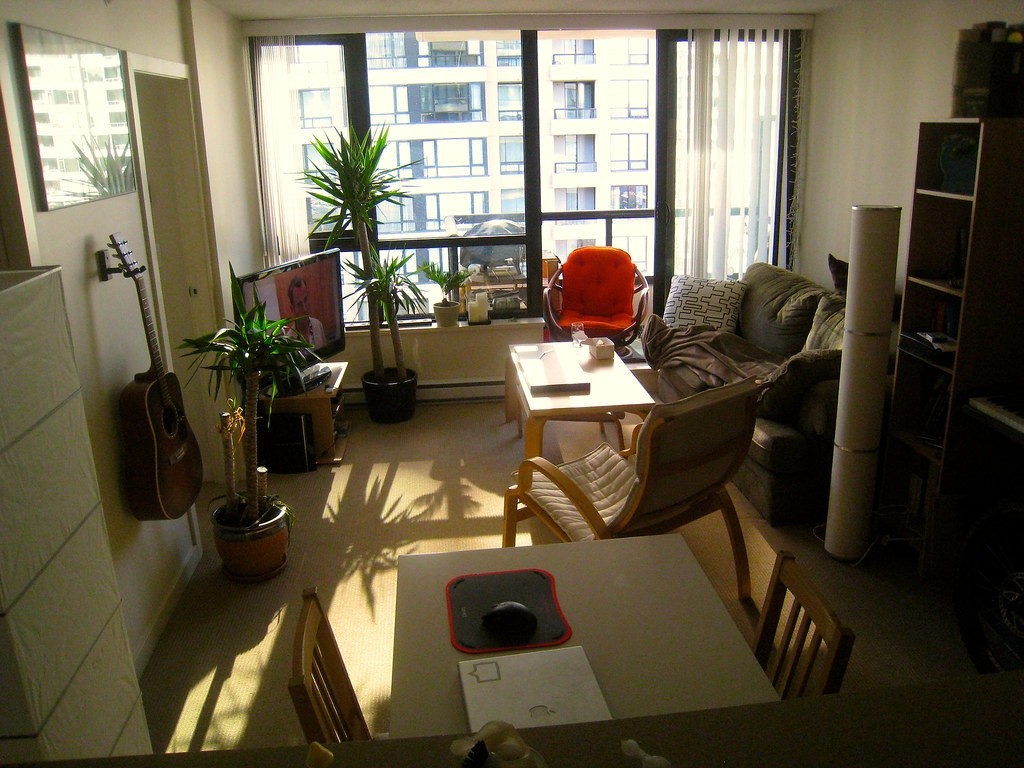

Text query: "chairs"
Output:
(502, 372), (759, 600)
(751, 549), (855, 703)
(542, 246), (650, 359)
(287, 587), (372, 744)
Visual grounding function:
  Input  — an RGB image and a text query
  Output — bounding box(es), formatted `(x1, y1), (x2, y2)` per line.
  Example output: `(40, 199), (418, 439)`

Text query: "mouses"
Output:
(481, 600), (538, 634)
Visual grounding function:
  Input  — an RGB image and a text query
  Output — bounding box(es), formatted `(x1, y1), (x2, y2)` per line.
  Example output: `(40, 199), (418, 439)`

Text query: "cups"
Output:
(571, 322), (584, 347)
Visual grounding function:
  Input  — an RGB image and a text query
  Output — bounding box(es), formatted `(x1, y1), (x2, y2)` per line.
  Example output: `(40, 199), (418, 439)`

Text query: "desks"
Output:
(505, 342), (657, 458)
(388, 533), (780, 744)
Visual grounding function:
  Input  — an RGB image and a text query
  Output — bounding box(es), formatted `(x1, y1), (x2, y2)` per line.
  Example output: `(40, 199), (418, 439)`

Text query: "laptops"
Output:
(458, 645), (612, 733)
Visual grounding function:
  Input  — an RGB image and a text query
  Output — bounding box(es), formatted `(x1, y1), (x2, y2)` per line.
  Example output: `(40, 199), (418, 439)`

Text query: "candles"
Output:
(467, 291), (489, 322)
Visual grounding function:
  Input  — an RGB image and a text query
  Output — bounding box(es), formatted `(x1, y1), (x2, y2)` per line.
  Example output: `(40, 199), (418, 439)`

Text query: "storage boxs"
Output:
(963, 40), (1024, 118)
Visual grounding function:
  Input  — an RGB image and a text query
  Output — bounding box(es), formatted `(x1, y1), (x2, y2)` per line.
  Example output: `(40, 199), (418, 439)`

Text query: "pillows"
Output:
(755, 348), (842, 421)
(663, 275), (750, 334)
(828, 253), (849, 298)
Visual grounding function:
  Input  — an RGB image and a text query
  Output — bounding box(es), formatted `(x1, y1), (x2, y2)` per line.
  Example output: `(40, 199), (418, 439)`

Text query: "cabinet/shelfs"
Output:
(880, 115), (1024, 579)
(0, 266), (155, 762)
(260, 362), (352, 466)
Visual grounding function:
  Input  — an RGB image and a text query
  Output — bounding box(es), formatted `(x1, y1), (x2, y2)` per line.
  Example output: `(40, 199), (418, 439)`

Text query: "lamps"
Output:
(823, 205), (903, 564)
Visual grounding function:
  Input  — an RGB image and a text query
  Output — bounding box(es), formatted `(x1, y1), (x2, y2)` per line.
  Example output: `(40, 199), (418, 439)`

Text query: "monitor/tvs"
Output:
(234, 248), (346, 396)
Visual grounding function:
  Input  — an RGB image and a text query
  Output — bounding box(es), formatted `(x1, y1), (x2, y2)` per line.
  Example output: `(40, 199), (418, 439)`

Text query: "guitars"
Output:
(108, 232), (205, 523)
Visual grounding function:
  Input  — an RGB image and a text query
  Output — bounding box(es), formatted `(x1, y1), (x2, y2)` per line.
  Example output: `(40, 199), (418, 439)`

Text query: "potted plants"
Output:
(418, 262), (475, 328)
(175, 259), (325, 583)
(296, 121), (429, 423)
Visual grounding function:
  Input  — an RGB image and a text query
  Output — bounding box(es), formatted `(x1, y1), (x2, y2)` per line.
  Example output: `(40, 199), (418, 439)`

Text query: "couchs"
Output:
(641, 263), (898, 528)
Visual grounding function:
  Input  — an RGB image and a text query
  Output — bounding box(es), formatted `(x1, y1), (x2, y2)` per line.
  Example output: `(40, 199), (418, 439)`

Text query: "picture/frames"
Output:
(14, 23), (139, 213)
